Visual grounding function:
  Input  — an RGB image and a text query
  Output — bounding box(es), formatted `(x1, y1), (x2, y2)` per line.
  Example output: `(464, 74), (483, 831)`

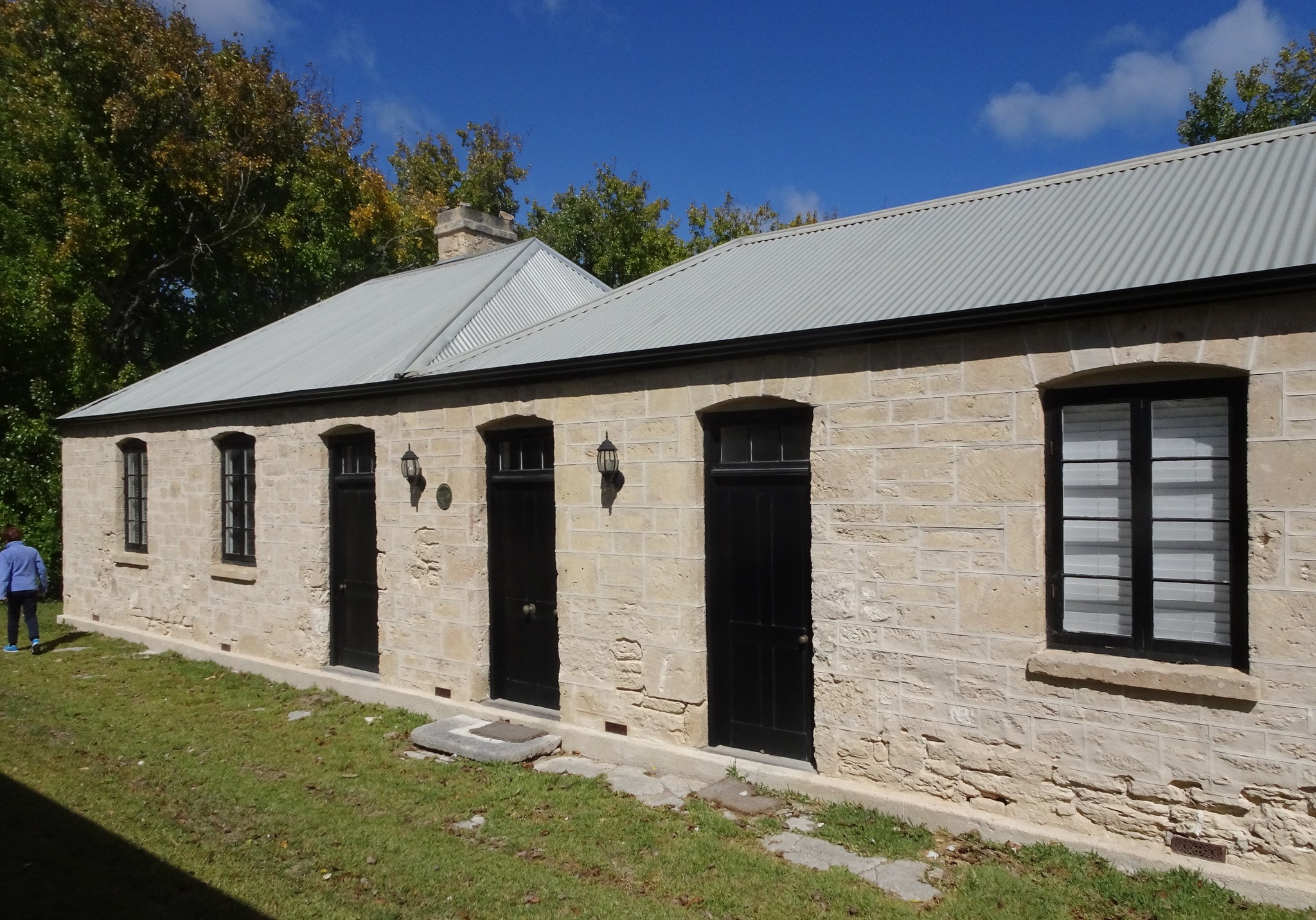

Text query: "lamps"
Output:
(597, 430), (624, 489)
(400, 443), (426, 493)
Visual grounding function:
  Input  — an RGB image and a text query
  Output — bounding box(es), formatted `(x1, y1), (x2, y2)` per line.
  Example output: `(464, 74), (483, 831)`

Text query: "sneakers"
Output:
(31, 639), (40, 654)
(4, 643), (19, 652)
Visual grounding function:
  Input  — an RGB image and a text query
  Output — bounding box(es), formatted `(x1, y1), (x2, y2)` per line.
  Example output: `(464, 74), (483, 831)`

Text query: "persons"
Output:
(0, 527), (48, 654)
